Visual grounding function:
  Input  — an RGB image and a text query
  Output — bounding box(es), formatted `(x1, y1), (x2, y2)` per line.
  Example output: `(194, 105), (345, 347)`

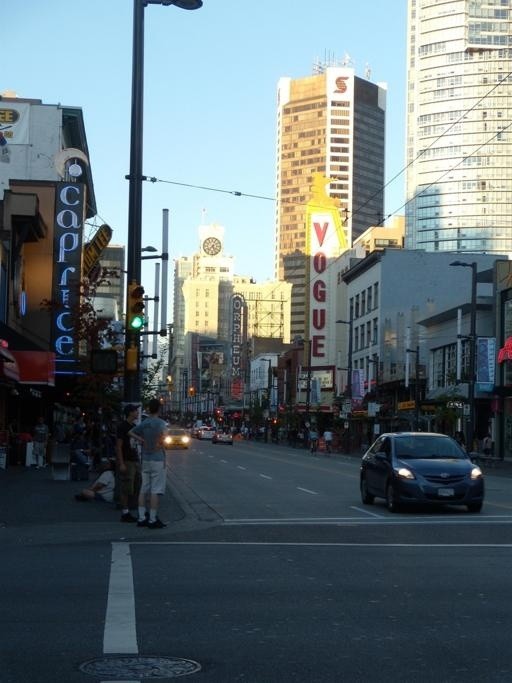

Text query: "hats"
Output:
(123, 402), (142, 414)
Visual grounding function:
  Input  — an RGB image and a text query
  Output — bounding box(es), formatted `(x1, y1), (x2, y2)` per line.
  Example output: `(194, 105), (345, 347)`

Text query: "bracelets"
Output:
(119, 462), (125, 465)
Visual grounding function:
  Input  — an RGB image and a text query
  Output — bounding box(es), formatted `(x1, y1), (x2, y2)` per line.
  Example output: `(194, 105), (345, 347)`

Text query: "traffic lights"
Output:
(89, 349), (117, 376)
(127, 282), (145, 331)
(189, 385), (195, 397)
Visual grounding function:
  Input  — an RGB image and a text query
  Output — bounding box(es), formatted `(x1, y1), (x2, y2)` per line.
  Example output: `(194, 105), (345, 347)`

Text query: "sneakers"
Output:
(120, 511), (169, 529)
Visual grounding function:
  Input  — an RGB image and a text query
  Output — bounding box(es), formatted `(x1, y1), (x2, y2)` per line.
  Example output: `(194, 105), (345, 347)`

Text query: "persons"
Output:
(482, 433), (493, 457)
(323, 428), (333, 448)
(76, 458), (116, 503)
(31, 415), (49, 471)
(128, 398), (167, 528)
(115, 403), (142, 522)
(310, 427), (319, 452)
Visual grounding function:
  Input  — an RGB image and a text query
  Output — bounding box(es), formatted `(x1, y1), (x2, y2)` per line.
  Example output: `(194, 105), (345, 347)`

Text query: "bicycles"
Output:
(308, 438), (333, 457)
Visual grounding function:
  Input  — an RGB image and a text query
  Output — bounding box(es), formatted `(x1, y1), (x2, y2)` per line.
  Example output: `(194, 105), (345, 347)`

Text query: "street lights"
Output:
(406, 346), (420, 424)
(259, 358), (271, 442)
(368, 356), (378, 387)
(298, 338), (311, 447)
(335, 319), (353, 454)
(449, 261), (477, 451)
(120, 1), (204, 464)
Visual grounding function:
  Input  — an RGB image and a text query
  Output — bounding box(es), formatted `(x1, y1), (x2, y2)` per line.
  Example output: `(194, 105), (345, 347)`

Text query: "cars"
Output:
(191, 423), (233, 445)
(359, 432), (484, 513)
(166, 428), (188, 449)
